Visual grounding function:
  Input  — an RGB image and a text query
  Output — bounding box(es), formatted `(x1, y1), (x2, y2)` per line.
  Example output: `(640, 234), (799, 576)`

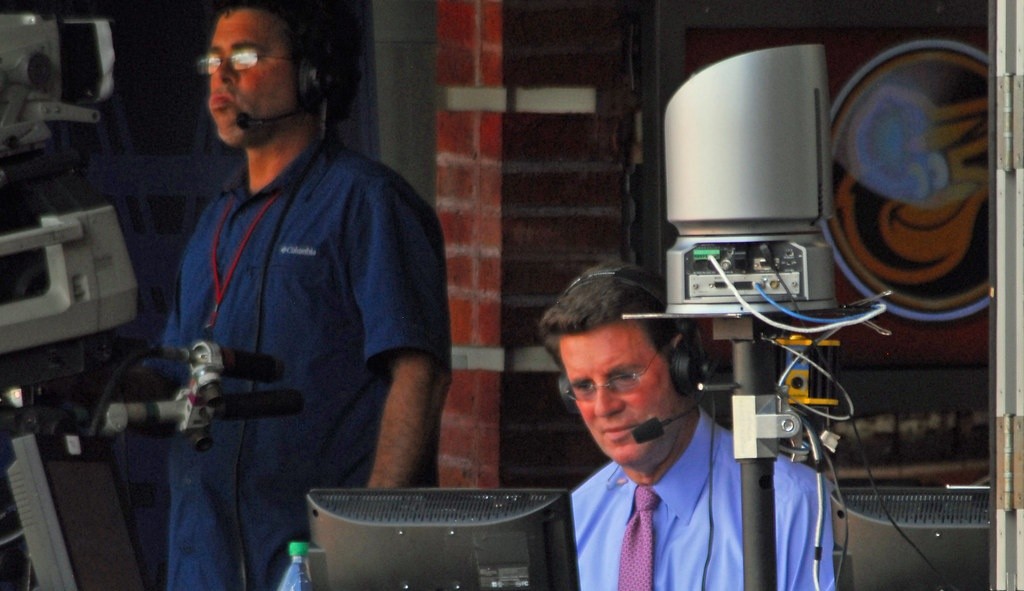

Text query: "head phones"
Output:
(236, 0), (361, 130)
(558, 271), (716, 443)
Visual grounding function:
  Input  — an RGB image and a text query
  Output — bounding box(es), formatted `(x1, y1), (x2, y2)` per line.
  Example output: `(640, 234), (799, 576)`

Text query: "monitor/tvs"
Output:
(830, 487), (990, 591)
(305, 487), (577, 591)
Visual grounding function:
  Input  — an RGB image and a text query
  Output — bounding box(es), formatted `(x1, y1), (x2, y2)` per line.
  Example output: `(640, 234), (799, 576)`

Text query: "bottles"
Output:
(278, 541), (314, 591)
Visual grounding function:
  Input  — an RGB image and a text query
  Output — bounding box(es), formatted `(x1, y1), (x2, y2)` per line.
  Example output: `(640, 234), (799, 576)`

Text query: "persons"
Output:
(540, 257), (836, 591)
(50, 0), (451, 591)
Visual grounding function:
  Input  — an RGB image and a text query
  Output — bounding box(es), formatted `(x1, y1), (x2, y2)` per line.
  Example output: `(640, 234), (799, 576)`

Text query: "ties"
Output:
(618, 486), (659, 591)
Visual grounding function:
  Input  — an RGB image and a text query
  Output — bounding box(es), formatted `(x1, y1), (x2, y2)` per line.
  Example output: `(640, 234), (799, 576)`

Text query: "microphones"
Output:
(630, 370), (713, 443)
(165, 342), (284, 383)
(95, 387), (304, 435)
(236, 105), (302, 130)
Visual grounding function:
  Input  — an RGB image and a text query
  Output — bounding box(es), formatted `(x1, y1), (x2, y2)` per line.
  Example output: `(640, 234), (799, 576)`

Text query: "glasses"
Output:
(197, 49), (294, 77)
(563, 347), (661, 400)
